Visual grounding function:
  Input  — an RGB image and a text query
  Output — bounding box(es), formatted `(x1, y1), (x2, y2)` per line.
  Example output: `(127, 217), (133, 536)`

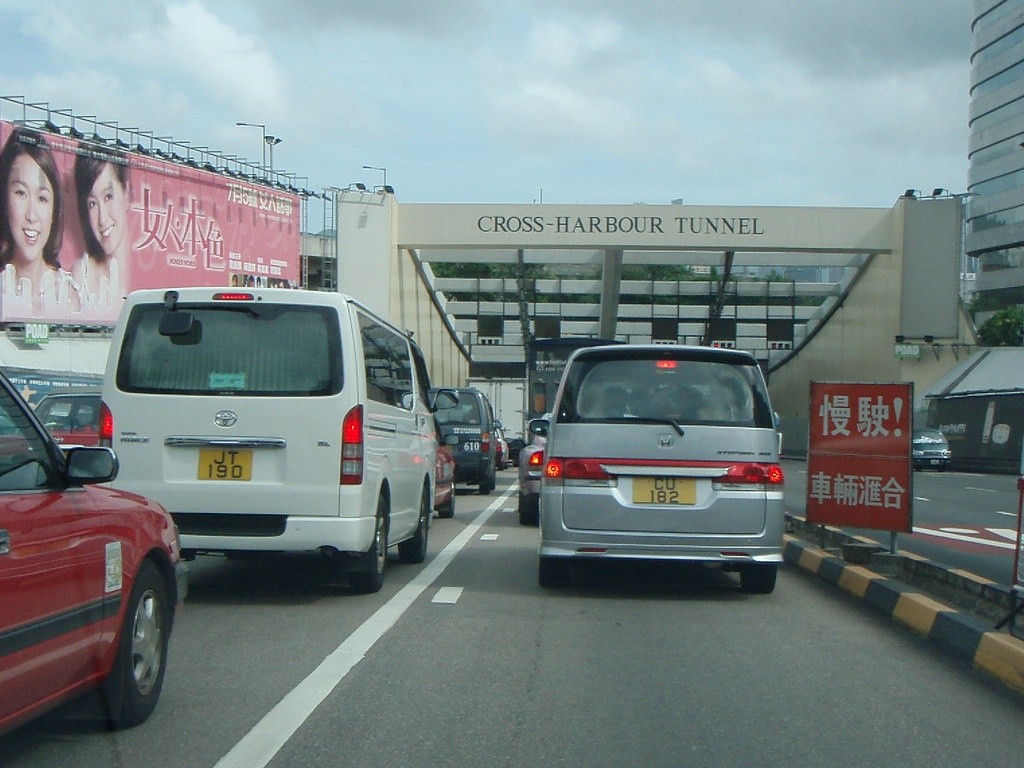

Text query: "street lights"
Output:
(236, 123), (265, 179)
(266, 134), (283, 179)
(363, 166), (386, 186)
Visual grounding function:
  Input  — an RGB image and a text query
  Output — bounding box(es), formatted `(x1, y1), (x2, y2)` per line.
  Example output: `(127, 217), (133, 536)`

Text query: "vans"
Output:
(468, 381), (529, 467)
(913, 427), (951, 472)
(97, 287), (460, 593)
(537, 345), (786, 592)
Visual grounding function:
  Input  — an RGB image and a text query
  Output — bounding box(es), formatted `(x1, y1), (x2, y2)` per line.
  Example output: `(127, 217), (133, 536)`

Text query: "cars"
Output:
(0, 372), (188, 738)
(510, 412), (553, 527)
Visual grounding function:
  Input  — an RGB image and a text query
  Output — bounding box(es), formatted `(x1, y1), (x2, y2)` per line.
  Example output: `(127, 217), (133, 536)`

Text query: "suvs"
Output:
(0, 391), (106, 461)
(428, 387), (524, 516)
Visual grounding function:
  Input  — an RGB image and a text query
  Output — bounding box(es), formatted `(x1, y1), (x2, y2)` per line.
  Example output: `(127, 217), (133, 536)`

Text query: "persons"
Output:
(0, 127), (79, 319)
(68, 144), (131, 321)
(232, 274), (290, 289)
